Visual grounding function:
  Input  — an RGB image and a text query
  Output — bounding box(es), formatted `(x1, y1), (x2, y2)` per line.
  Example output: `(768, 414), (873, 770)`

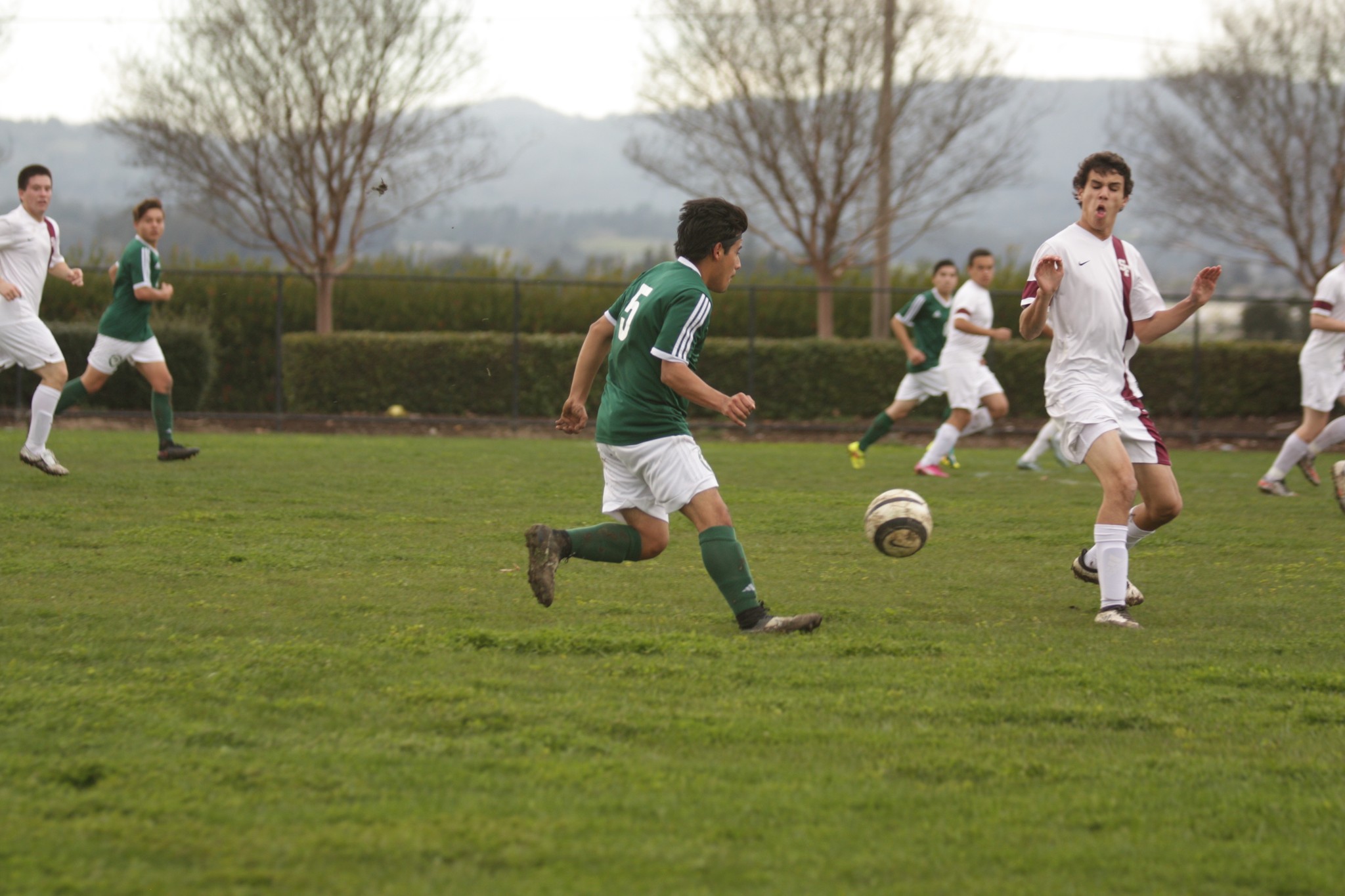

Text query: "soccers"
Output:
(864, 489), (933, 558)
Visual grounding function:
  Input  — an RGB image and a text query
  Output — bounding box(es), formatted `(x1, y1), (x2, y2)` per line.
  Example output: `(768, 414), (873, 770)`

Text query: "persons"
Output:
(848, 260), (961, 470)
(27, 199), (200, 460)
(1019, 152), (1221, 628)
(1257, 260), (1345, 514)
(1016, 302), (1056, 471)
(0, 165), (84, 475)
(913, 249), (1012, 477)
(525, 197), (822, 633)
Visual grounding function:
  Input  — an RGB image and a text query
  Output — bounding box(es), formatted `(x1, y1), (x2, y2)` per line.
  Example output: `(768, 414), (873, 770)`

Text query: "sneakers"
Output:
(1070, 548), (1144, 606)
(1331, 460), (1345, 512)
(1094, 607), (1140, 628)
(525, 524), (561, 608)
(1297, 454), (1321, 487)
(20, 443), (71, 475)
(157, 444), (199, 461)
(747, 612), (822, 633)
(926, 440), (960, 469)
(1257, 476), (1298, 496)
(914, 460), (949, 477)
(848, 440), (866, 469)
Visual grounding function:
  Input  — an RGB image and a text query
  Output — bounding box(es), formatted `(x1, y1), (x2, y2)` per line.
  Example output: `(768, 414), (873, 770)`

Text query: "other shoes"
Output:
(1048, 437), (1072, 468)
(1014, 458), (1042, 472)
